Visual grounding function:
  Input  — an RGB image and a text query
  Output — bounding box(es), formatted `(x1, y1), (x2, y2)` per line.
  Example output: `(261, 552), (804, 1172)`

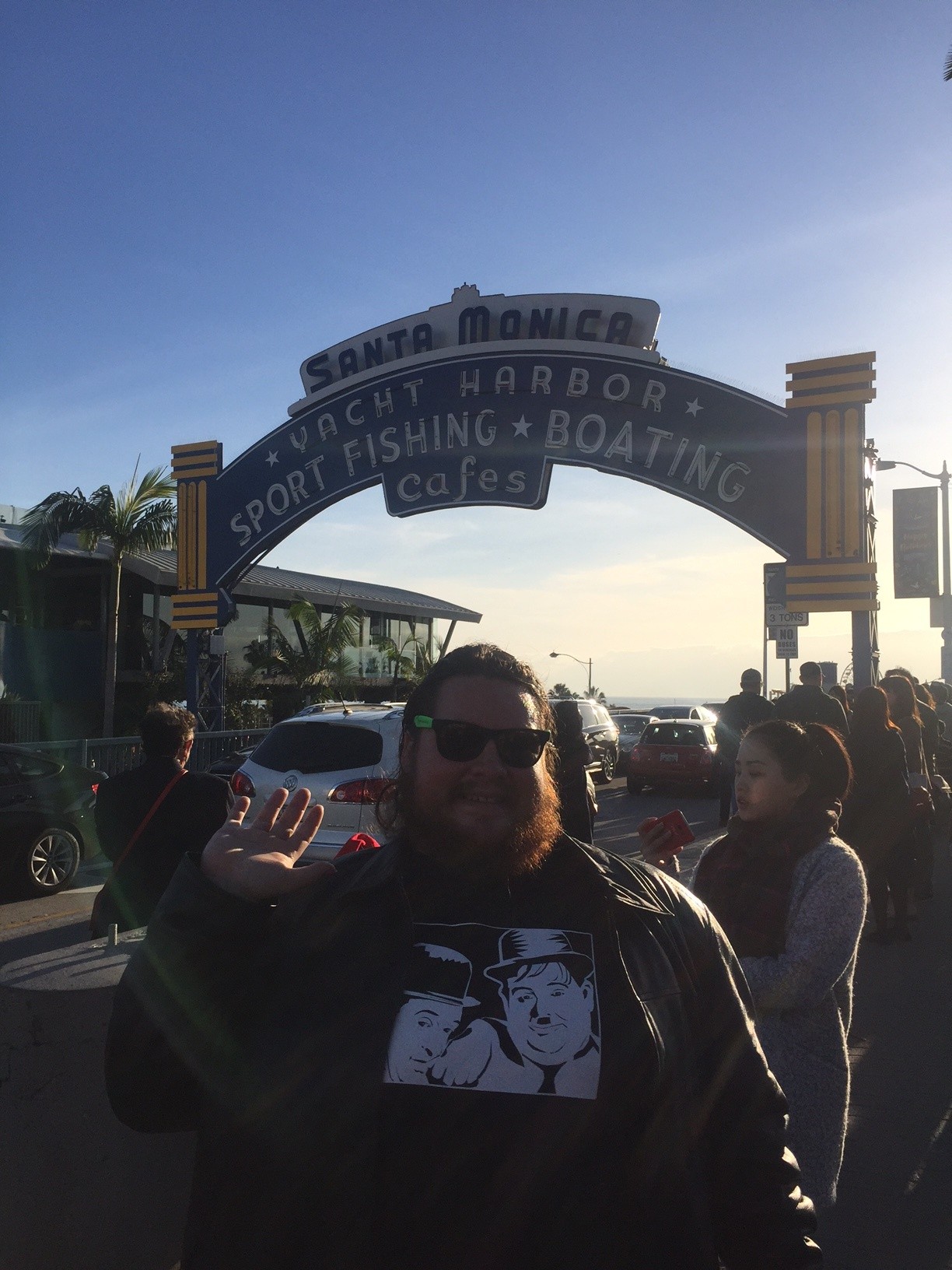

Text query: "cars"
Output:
(285, 702), (376, 720)
(702, 702), (728, 716)
(627, 720), (716, 792)
(0, 741), (108, 894)
(607, 706), (653, 716)
(648, 705), (720, 727)
(227, 688), (407, 869)
(605, 713), (659, 770)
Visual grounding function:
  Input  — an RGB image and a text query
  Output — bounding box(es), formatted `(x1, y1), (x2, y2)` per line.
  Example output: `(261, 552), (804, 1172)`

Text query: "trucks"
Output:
(540, 697), (621, 784)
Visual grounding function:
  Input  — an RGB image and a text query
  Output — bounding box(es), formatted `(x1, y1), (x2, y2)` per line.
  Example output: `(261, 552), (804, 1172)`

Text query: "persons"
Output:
(104, 643), (826, 1270)
(555, 701), (598, 844)
(96, 700), (233, 926)
(711, 663), (952, 943)
(637, 719), (872, 1228)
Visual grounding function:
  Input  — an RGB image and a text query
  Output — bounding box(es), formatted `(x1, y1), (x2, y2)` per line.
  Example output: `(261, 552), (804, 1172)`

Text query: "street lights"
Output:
(549, 652), (593, 698)
(874, 458), (952, 686)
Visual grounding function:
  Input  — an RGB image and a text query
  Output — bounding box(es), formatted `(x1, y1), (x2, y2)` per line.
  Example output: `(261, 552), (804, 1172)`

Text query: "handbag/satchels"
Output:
(89, 888), (138, 940)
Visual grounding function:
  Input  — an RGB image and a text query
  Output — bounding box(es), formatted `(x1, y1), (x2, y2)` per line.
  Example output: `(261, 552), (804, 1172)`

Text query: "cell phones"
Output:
(643, 809), (695, 853)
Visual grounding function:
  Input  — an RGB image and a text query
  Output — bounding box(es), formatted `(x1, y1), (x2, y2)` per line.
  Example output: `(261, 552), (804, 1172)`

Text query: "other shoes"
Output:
(864, 889), (935, 945)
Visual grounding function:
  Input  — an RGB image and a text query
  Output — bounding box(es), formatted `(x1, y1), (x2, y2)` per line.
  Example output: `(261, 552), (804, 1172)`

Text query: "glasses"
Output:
(413, 713), (552, 768)
